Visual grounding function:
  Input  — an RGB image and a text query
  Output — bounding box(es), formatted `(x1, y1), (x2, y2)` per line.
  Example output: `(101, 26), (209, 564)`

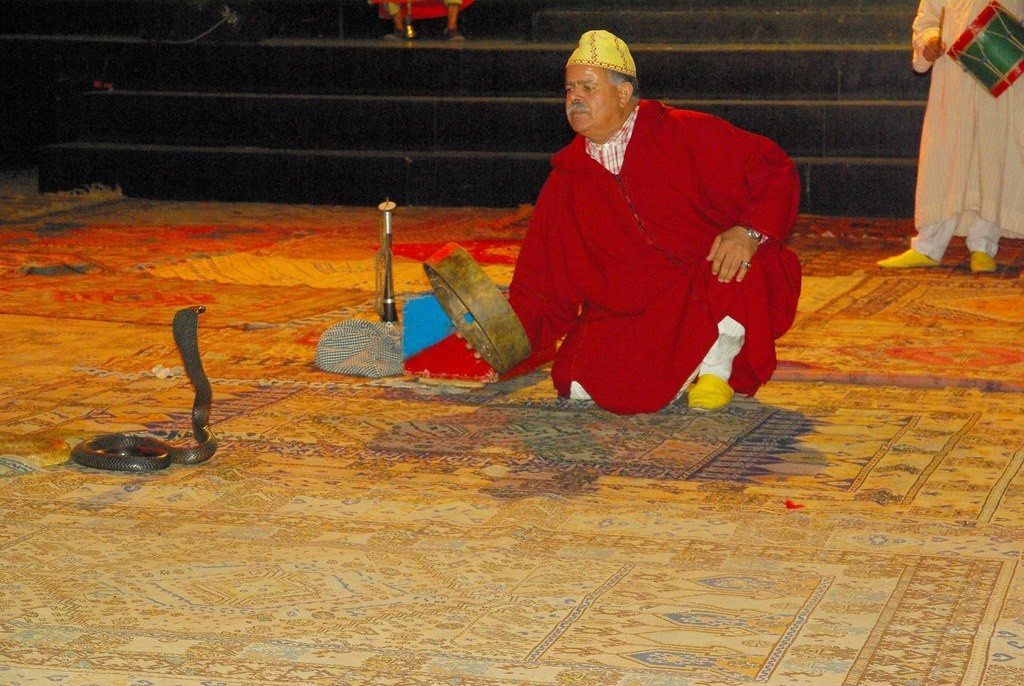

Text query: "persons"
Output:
(456, 29), (801, 415)
(877, 0), (1024, 274)
(369, 0), (475, 42)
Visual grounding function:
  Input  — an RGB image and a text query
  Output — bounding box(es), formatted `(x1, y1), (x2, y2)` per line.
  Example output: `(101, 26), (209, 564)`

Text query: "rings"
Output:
(744, 261), (751, 269)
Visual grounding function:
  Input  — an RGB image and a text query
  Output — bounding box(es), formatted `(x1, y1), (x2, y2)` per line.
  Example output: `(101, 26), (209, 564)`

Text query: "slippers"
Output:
(877, 248), (939, 268)
(689, 375), (734, 413)
(970, 250), (996, 272)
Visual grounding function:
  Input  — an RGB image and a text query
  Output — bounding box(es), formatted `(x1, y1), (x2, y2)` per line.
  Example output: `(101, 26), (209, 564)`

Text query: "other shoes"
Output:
(384, 29), (403, 43)
(445, 28), (465, 42)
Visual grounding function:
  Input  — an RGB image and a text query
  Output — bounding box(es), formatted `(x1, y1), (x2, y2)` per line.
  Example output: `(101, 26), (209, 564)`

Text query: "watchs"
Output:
(749, 227), (762, 243)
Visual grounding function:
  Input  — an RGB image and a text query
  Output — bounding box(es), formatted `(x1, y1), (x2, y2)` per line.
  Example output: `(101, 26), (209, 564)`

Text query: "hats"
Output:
(566, 30), (636, 77)
